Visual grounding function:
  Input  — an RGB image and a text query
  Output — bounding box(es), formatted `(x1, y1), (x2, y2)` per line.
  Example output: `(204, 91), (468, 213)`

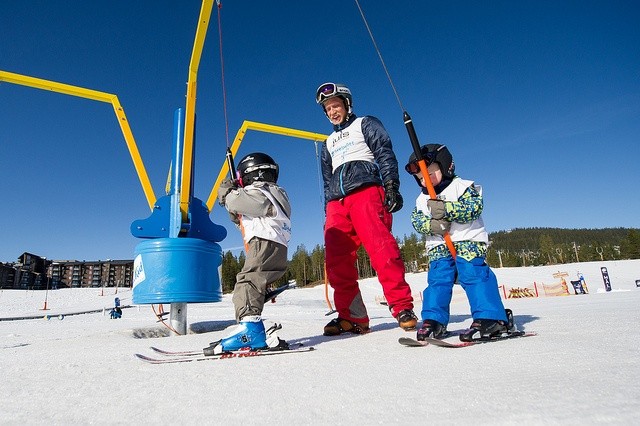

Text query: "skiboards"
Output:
(399, 330), (537, 347)
(135, 343), (315, 365)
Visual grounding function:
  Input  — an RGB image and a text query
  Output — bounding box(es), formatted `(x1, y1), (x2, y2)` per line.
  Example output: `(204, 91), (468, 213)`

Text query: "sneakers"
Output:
(459, 319), (508, 341)
(417, 319), (448, 340)
(219, 316), (268, 351)
(396, 311), (417, 330)
(324, 317), (369, 336)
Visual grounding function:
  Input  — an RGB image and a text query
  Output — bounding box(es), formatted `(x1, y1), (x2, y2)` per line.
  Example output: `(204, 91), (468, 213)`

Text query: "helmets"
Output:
(316, 82), (352, 121)
(405, 144), (455, 187)
(236, 153), (279, 184)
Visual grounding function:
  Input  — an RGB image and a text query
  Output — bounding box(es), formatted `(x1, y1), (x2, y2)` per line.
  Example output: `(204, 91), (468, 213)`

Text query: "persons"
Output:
(314, 83), (417, 334)
(109, 308), (116, 319)
(406, 142), (510, 340)
(203, 153), (291, 355)
(114, 307), (123, 320)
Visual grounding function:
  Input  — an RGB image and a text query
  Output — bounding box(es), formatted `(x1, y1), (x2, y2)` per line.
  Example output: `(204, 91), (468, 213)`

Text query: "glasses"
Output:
(405, 145), (446, 175)
(317, 83), (351, 103)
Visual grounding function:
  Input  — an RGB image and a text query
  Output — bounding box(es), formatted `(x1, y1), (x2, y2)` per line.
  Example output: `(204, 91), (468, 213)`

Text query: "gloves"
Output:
(217, 177), (239, 207)
(429, 219), (451, 235)
(382, 185), (403, 212)
(426, 198), (446, 219)
